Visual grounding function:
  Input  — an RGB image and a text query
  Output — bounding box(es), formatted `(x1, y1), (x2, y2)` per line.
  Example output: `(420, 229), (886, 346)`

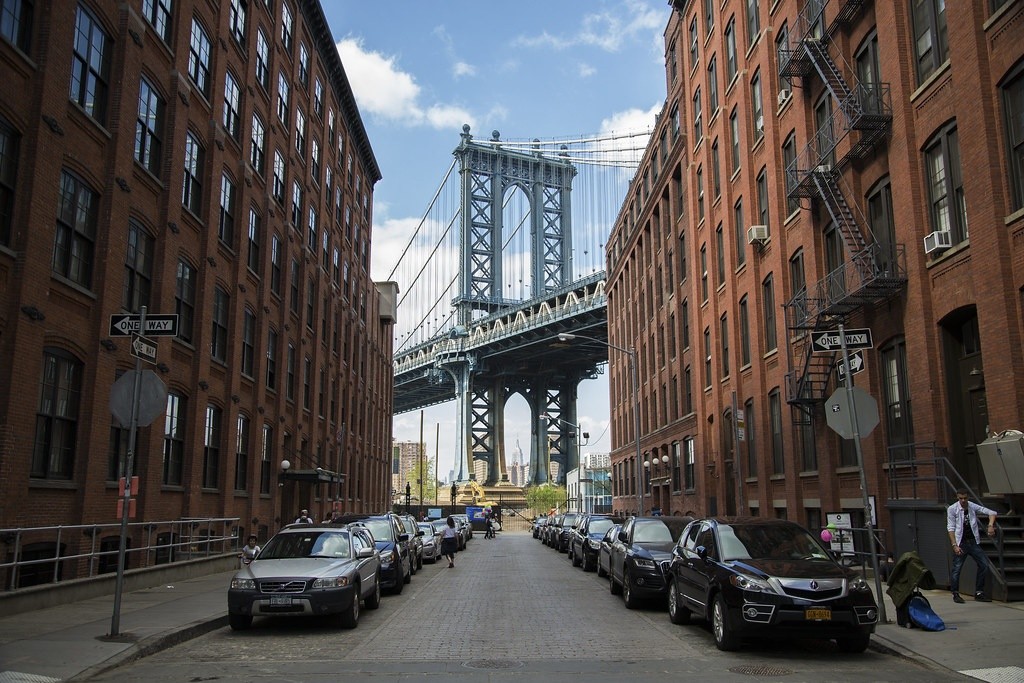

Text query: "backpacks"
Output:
(299, 516), (309, 523)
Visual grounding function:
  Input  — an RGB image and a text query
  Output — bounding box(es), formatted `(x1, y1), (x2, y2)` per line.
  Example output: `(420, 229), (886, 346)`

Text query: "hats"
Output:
(301, 510), (309, 515)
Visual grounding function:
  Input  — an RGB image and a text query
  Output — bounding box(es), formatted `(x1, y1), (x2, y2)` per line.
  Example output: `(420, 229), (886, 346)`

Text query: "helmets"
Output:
(247, 533), (257, 543)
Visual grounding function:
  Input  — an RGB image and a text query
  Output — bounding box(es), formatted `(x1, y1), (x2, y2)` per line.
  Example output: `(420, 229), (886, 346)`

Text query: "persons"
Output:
(294, 510), (313, 524)
(238, 533), (260, 568)
(421, 514), (428, 522)
(947, 488), (997, 603)
(816, 519), (832, 549)
(439, 517), (457, 568)
(484, 507), (495, 540)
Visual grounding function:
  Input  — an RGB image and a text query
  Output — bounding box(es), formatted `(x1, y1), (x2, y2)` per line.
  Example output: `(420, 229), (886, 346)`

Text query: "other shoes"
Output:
(953, 596), (966, 603)
(449, 563), (454, 568)
(974, 593), (992, 602)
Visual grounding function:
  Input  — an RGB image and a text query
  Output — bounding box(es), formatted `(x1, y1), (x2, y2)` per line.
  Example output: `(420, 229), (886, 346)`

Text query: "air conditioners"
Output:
(747, 225), (767, 248)
(924, 230), (953, 255)
(757, 115), (764, 133)
(777, 88), (791, 107)
(814, 165), (831, 172)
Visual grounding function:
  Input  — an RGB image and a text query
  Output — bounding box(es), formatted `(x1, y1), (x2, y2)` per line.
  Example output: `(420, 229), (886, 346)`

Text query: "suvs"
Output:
(227, 522), (382, 631)
(609, 515), (697, 610)
(395, 513), (424, 575)
(326, 511), (414, 595)
(667, 515), (880, 654)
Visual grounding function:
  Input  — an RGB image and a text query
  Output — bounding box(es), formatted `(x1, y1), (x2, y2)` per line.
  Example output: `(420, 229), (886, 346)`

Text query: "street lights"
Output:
(557, 331), (645, 520)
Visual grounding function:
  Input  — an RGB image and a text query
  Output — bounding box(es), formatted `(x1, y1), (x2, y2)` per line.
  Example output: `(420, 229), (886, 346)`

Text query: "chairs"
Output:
(323, 534), (347, 555)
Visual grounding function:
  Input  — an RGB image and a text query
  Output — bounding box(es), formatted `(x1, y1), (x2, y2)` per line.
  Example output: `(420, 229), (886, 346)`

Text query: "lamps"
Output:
(607, 472), (613, 482)
(707, 456), (738, 479)
(278, 460), (291, 486)
(644, 455), (671, 475)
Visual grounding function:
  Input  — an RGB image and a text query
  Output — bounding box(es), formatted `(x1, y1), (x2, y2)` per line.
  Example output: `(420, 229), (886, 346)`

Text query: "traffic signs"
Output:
(108, 314), (179, 337)
(810, 328), (874, 353)
(130, 331), (158, 365)
(836, 349), (865, 382)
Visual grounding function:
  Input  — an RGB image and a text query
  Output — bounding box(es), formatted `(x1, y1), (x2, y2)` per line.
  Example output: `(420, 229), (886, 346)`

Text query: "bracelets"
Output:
(987, 525), (994, 527)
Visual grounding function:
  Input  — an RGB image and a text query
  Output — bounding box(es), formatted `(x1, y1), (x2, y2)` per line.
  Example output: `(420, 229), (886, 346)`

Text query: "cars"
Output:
(416, 521), (443, 565)
(596, 523), (623, 578)
(432, 514), (474, 553)
(531, 511), (619, 572)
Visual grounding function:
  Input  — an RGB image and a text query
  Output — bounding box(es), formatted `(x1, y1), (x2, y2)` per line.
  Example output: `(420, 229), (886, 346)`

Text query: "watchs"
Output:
(951, 543), (957, 547)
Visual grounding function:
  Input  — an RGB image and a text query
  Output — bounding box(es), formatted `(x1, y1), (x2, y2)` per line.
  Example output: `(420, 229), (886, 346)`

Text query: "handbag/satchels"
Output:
(909, 596), (946, 631)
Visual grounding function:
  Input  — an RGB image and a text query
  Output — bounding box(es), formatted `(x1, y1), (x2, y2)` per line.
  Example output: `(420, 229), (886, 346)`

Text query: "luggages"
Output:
(895, 585), (923, 629)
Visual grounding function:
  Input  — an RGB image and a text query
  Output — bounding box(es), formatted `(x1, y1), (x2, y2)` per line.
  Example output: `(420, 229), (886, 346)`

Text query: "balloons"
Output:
(821, 529), (831, 542)
(481, 503), (492, 516)
(827, 523), (835, 532)
